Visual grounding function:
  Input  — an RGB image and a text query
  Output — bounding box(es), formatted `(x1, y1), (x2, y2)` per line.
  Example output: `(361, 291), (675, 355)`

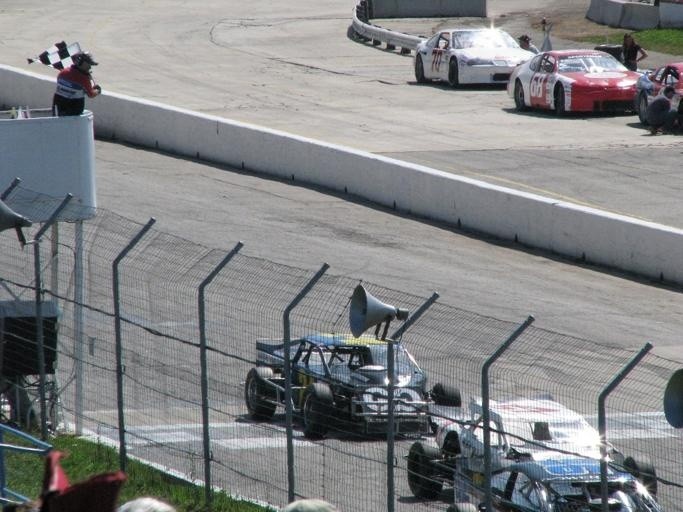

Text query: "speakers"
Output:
(0, 300), (58, 375)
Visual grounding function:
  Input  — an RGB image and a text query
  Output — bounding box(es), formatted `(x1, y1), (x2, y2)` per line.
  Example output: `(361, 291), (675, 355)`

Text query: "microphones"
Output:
(81, 69), (92, 74)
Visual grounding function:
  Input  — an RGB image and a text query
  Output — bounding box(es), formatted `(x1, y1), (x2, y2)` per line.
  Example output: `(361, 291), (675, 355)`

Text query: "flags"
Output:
(23, 39), (84, 72)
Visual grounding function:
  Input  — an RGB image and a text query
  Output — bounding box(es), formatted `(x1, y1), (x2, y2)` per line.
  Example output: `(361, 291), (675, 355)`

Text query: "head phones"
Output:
(76, 56), (84, 65)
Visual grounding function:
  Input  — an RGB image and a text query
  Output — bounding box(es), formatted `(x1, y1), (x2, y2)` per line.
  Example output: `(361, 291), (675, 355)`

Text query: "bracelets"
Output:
(638, 58), (641, 62)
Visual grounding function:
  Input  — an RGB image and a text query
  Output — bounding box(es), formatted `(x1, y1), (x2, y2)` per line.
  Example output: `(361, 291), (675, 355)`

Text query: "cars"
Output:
(636, 62), (683, 127)
(414, 28), (536, 88)
(507, 49), (643, 116)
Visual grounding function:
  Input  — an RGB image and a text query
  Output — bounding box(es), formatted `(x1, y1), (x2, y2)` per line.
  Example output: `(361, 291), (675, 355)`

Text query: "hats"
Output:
(517, 35), (530, 41)
(70, 52), (98, 66)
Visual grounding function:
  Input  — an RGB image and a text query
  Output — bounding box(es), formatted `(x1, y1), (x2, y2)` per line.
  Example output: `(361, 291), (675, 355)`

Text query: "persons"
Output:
(643, 85), (678, 135)
(50, 49), (102, 117)
(615, 32), (649, 73)
(516, 35), (540, 55)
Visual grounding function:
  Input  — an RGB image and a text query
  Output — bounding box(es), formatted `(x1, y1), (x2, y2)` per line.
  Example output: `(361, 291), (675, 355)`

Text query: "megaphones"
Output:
(0, 200), (32, 232)
(663, 368), (683, 428)
(349, 284), (409, 337)
